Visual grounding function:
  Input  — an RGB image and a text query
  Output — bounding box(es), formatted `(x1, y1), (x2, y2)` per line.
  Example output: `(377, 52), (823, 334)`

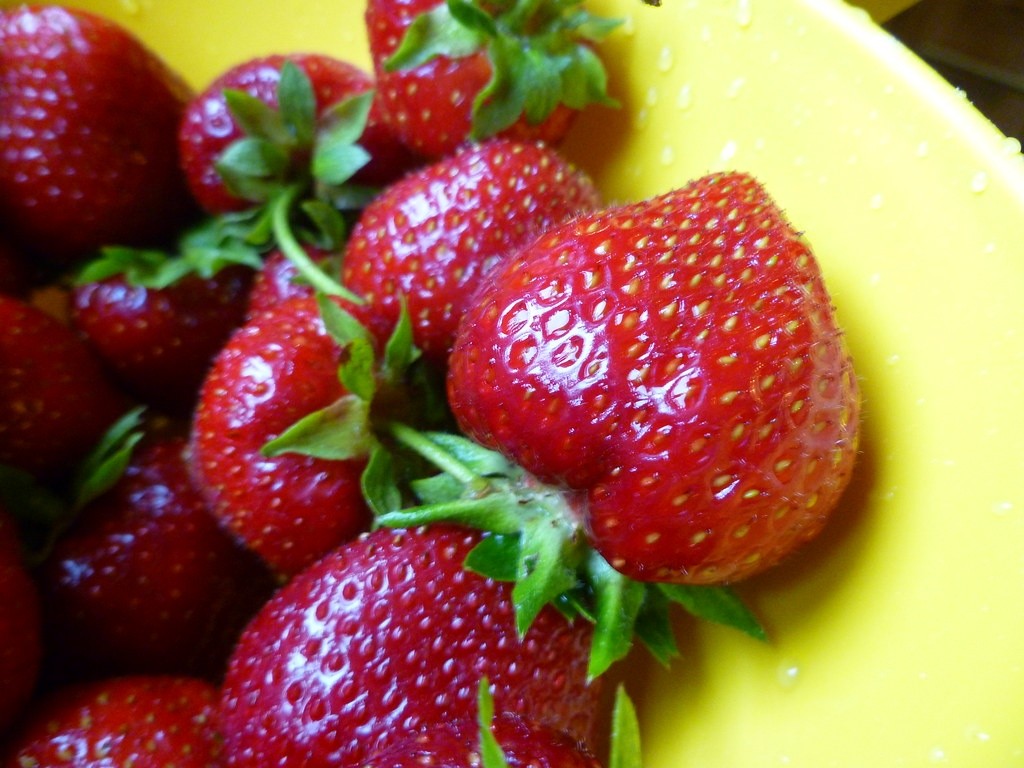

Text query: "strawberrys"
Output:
(0, 0), (864, 767)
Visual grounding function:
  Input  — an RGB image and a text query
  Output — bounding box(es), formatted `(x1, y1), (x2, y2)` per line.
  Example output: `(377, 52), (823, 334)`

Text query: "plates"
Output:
(1, 2), (1021, 768)
